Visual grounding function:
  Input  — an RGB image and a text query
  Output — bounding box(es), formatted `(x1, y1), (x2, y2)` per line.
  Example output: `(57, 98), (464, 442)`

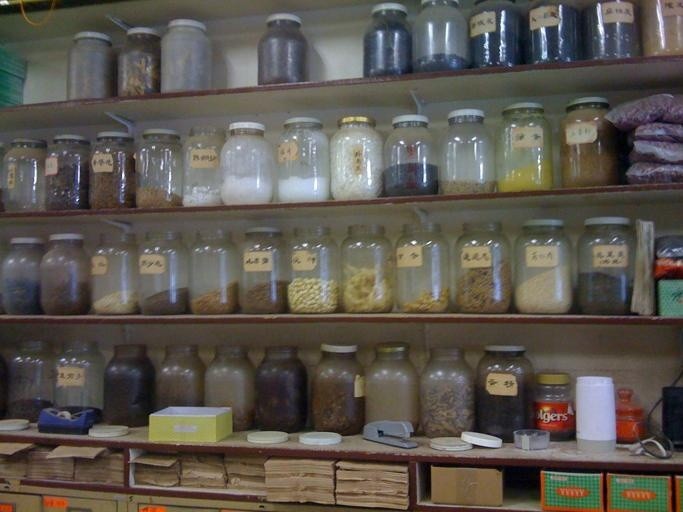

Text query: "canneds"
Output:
(1, 94), (611, 217)
(3, 212), (636, 318)
(362, 0), (683, 79)
(66, 30), (111, 102)
(616, 404), (647, 444)
(654, 231), (682, 319)
(4, 340), (531, 442)
(117, 25), (160, 100)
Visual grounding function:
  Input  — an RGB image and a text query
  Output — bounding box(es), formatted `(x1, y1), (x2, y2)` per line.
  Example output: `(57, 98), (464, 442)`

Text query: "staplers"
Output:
(361, 416), (420, 452)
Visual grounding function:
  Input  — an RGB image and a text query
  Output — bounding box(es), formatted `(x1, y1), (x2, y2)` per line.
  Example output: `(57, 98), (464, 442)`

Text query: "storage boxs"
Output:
(673, 476), (683, 511)
(603, 472), (674, 511)
(428, 461), (507, 507)
(539, 466), (604, 512)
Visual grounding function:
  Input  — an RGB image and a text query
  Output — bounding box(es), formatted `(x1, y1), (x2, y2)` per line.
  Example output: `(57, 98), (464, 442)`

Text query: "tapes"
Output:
(53, 409), (95, 420)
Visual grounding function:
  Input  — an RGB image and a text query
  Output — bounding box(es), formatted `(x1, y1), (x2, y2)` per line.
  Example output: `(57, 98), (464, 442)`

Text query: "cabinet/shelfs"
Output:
(129, 495), (398, 512)
(0, 1), (682, 512)
(0, 485), (128, 512)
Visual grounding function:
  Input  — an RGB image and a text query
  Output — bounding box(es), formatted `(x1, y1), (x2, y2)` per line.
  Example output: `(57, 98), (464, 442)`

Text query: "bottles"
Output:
(198, 344), (256, 429)
(237, 225), (285, 314)
(573, 217), (635, 313)
(419, 343), (475, 442)
(44, 134), (88, 210)
(340, 224), (392, 312)
(113, 27), (158, 98)
(0, 238), (40, 316)
(133, 128), (179, 208)
(468, 0), (522, 71)
(38, 233), (88, 316)
(394, 221), (453, 314)
(456, 222), (511, 314)
(411, 1), (471, 75)
(3, 138), (43, 211)
(384, 114), (436, 197)
(639, 1), (682, 62)
(330, 114), (382, 200)
(154, 340), (206, 422)
(220, 120), (274, 205)
(559, 96), (619, 190)
(525, 0), (578, 65)
(585, 2), (636, 63)
(510, 218), (573, 314)
(65, 30), (117, 98)
(358, 340), (421, 435)
(285, 223), (342, 316)
(255, 346), (307, 431)
(88, 232), (138, 315)
(187, 229), (237, 314)
(3, 338), (151, 427)
(496, 102), (555, 191)
(361, 2), (413, 78)
(438, 108), (494, 194)
(181, 122), (221, 208)
(137, 228), (189, 314)
(475, 344), (535, 440)
(613, 389), (644, 443)
(88, 131), (136, 209)
(276, 117), (328, 204)
(307, 341), (366, 435)
